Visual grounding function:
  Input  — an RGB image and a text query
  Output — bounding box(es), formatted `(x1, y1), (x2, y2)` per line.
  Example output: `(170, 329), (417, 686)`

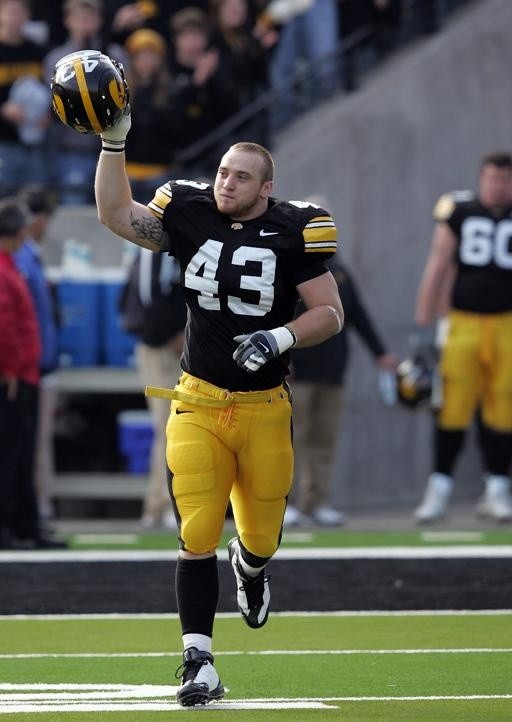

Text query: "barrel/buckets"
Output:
(101, 269), (142, 367)
(42, 263), (102, 370)
(120, 410), (153, 472)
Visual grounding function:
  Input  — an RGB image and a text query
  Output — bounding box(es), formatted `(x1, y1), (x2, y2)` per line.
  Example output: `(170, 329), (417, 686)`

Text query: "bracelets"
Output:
(282, 326), (298, 347)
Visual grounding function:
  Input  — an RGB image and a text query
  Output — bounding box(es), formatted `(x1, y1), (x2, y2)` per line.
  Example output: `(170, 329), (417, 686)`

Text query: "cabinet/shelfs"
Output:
(36, 365), (153, 520)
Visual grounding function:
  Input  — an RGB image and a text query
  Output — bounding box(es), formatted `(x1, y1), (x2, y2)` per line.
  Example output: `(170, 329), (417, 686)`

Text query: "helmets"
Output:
(396, 357), (433, 407)
(51, 51), (128, 136)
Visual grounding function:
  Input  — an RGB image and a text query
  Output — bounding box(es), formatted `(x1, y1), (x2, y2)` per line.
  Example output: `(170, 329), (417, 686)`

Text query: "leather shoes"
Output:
(2, 533), (70, 552)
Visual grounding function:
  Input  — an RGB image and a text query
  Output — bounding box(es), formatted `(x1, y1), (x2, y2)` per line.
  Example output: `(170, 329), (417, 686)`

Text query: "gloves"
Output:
(100, 107), (131, 154)
(379, 370), (396, 408)
(233, 327), (298, 373)
(410, 325), (440, 370)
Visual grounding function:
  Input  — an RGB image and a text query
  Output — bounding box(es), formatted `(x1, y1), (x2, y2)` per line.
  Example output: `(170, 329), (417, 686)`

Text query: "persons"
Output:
(1, 0), (465, 205)
(403, 153), (511, 524)
(280, 194), (401, 529)
(91, 101), (347, 709)
(114, 242), (190, 529)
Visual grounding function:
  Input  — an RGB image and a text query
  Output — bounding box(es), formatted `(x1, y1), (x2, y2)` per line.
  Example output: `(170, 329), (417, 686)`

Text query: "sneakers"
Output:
(414, 482), (453, 523)
(228, 537), (271, 629)
(475, 495), (512, 523)
(283, 506), (307, 528)
(311, 507), (346, 526)
(177, 648), (225, 707)
